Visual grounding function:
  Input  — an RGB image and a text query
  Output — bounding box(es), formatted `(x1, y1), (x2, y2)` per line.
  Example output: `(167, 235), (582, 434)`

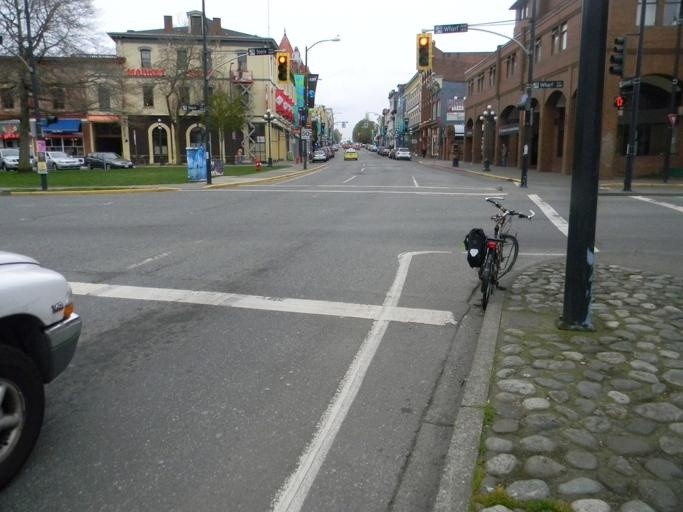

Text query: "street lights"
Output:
(262, 108), (275, 166)
(328, 111), (347, 145)
(302, 33), (340, 169)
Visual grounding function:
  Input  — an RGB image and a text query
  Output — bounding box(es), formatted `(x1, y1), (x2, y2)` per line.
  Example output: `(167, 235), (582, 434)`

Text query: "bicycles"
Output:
(474, 198), (535, 306)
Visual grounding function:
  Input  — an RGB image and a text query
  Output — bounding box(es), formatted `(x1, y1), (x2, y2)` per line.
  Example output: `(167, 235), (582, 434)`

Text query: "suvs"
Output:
(0, 250), (80, 492)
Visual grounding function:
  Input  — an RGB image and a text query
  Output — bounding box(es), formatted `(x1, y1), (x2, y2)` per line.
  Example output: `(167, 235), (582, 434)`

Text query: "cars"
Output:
(0, 148), (134, 174)
(310, 141), (411, 163)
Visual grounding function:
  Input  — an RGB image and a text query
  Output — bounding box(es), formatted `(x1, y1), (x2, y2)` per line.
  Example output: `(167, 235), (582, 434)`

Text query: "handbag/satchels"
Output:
(464, 227), (487, 268)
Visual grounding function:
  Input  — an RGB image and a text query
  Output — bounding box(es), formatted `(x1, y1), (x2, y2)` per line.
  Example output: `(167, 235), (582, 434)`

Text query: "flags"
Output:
(315, 122), (332, 138)
(300, 107), (308, 129)
(292, 106), (298, 130)
(294, 75), (305, 108)
(306, 74), (318, 109)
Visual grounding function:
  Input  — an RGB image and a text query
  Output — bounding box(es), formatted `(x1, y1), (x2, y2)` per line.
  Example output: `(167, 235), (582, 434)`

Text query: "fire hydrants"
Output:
(255, 159), (261, 172)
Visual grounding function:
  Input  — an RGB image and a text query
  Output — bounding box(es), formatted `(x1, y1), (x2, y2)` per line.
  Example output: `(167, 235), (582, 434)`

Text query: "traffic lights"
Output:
(608, 36), (624, 75)
(276, 52), (290, 83)
(614, 95), (624, 109)
(515, 101), (524, 112)
(417, 33), (432, 71)
(45, 113), (57, 123)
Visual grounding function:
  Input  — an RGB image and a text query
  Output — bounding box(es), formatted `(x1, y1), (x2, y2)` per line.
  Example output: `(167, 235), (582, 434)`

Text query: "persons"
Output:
(237, 146), (242, 163)
(500, 143), (508, 166)
(421, 147), (426, 158)
(293, 154), (301, 170)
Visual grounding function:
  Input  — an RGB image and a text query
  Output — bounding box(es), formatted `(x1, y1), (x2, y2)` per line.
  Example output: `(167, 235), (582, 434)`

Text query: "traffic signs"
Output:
(533, 81), (563, 88)
(182, 105), (204, 111)
(434, 24), (467, 34)
(300, 128), (312, 140)
(245, 48), (274, 56)
